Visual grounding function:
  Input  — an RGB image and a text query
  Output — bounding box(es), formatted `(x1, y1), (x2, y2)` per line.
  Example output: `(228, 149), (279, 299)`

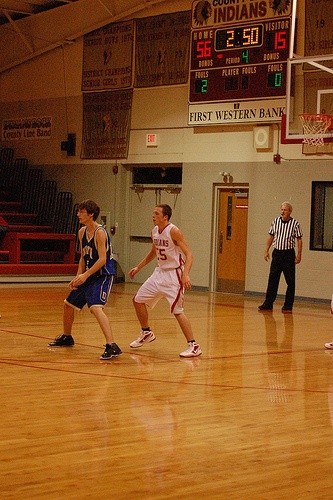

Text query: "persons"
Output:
(128, 204), (202, 357)
(258, 202), (303, 311)
(325, 296), (333, 349)
(47, 199), (123, 359)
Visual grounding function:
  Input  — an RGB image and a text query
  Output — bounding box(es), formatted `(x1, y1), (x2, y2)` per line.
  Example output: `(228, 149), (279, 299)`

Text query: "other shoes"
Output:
(258, 303), (273, 309)
(282, 305), (292, 310)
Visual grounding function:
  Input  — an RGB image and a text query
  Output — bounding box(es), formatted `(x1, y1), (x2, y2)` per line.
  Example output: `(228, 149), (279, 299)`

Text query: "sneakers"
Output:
(98, 343), (122, 360)
(324, 342), (333, 350)
(129, 330), (156, 348)
(179, 341), (202, 357)
(48, 333), (74, 347)
